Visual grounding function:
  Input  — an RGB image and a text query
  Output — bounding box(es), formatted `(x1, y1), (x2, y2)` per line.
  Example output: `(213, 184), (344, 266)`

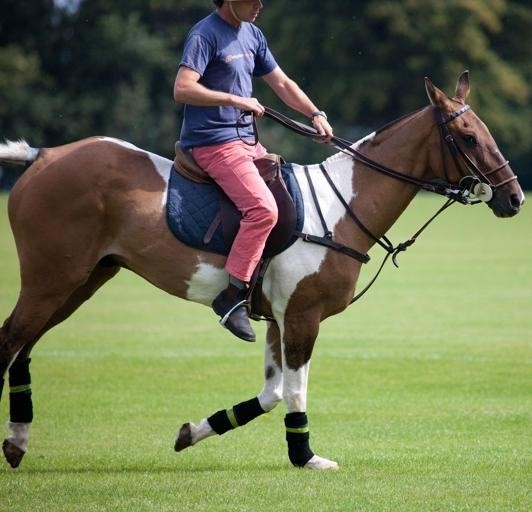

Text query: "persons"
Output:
(174, 0), (334, 341)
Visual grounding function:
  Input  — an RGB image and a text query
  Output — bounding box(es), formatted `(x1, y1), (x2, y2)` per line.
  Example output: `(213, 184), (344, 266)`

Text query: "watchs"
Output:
(311, 110), (328, 121)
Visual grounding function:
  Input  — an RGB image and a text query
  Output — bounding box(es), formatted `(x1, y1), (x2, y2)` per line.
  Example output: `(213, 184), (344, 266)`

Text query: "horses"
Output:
(0, 69), (526, 470)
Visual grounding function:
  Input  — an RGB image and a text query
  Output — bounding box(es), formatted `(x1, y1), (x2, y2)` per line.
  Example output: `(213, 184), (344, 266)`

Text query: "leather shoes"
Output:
(212, 290), (255, 343)
